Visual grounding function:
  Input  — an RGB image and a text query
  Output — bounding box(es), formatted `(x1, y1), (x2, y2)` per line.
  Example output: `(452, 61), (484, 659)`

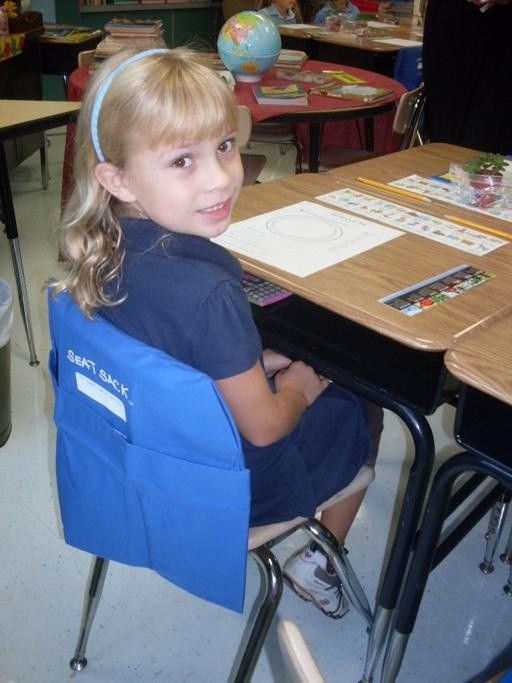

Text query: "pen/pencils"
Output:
(441, 214), (512, 240)
(355, 176), (433, 203)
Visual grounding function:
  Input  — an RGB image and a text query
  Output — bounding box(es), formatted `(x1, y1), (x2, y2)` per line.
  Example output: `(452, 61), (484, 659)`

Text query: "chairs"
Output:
(46, 284), (374, 683)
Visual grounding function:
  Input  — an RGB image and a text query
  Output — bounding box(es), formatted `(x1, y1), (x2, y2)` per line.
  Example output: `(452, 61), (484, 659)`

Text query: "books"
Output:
(272, 48), (309, 70)
(85, 16), (169, 72)
(249, 81), (313, 104)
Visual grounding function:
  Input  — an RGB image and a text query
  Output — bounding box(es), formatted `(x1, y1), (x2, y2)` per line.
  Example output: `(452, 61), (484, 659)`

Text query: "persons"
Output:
(314, 0), (360, 23)
(259, 0), (297, 27)
(39, 35), (382, 617)
(418, 0), (512, 161)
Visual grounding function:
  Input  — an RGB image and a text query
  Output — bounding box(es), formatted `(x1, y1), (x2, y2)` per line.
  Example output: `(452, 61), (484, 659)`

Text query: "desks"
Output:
(275, 20), (425, 176)
(0, 97), (89, 367)
(208, 143), (512, 678)
(59, 52), (409, 257)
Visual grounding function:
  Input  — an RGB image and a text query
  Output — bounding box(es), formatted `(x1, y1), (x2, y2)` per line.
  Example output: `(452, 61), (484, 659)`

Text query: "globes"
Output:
(217, 11), (282, 83)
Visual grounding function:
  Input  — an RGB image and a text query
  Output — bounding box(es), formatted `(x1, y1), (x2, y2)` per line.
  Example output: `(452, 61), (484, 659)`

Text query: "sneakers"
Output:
(281, 536), (351, 619)
